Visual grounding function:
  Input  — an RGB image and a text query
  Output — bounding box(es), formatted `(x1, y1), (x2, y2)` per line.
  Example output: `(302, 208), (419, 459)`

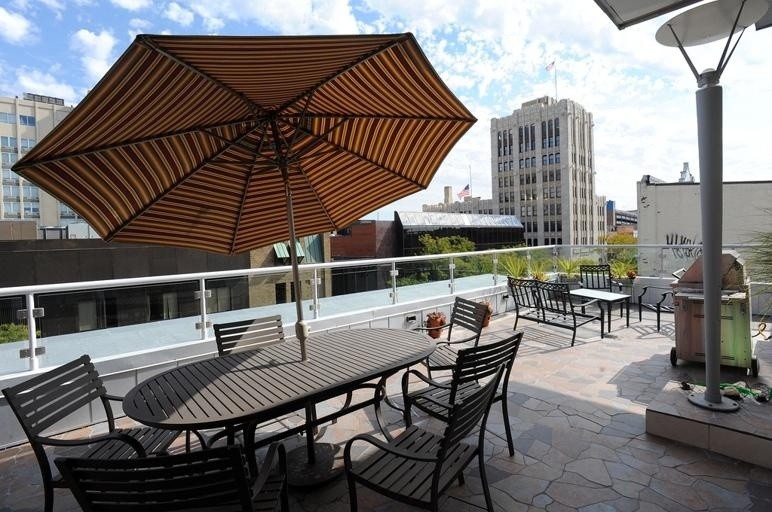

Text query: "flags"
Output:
(458, 184), (469, 200)
(545, 61), (555, 71)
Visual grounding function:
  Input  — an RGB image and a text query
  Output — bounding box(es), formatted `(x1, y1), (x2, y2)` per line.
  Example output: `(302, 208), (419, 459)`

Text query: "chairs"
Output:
(54, 443), (288, 511)
(402, 333), (524, 486)
(509, 265), (687, 347)
(2, 354), (208, 511)
(213, 314), (319, 446)
(405, 296), (490, 386)
(344, 363), (505, 510)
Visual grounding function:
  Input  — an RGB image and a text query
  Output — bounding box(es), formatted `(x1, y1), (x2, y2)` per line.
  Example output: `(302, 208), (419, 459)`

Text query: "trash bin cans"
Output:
(670, 251), (764, 372)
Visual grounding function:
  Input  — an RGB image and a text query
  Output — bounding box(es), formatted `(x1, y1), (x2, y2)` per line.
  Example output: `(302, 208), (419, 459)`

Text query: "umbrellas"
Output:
(11, 32), (477, 462)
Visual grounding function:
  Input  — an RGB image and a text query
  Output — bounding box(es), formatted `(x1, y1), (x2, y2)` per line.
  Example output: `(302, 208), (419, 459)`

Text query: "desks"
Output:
(123, 327), (438, 487)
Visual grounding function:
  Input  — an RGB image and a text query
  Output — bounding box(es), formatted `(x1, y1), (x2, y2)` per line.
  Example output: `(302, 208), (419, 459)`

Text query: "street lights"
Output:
(653, 0), (771, 416)
